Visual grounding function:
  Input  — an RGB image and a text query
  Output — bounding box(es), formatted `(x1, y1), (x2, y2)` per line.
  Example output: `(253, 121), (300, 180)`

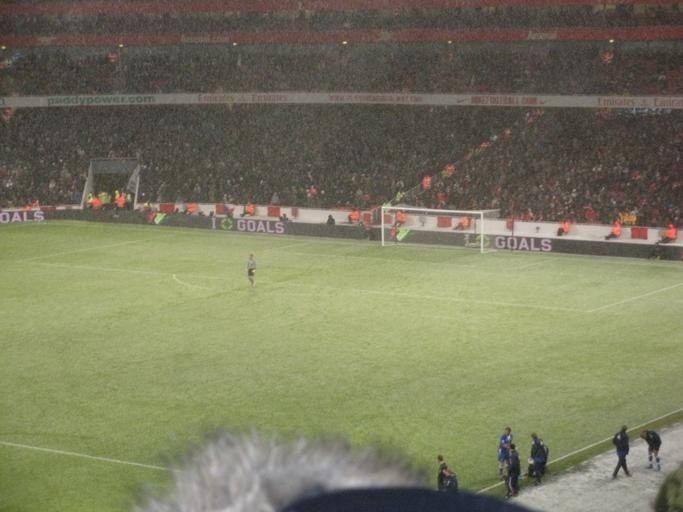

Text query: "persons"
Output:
(497, 426), (550, 499)
(640, 429), (662, 471)
(1, 4), (683, 248)
(128, 423), (430, 512)
(247, 252), (257, 287)
(441, 467), (457, 492)
(437, 455), (447, 490)
(612, 424), (630, 477)
